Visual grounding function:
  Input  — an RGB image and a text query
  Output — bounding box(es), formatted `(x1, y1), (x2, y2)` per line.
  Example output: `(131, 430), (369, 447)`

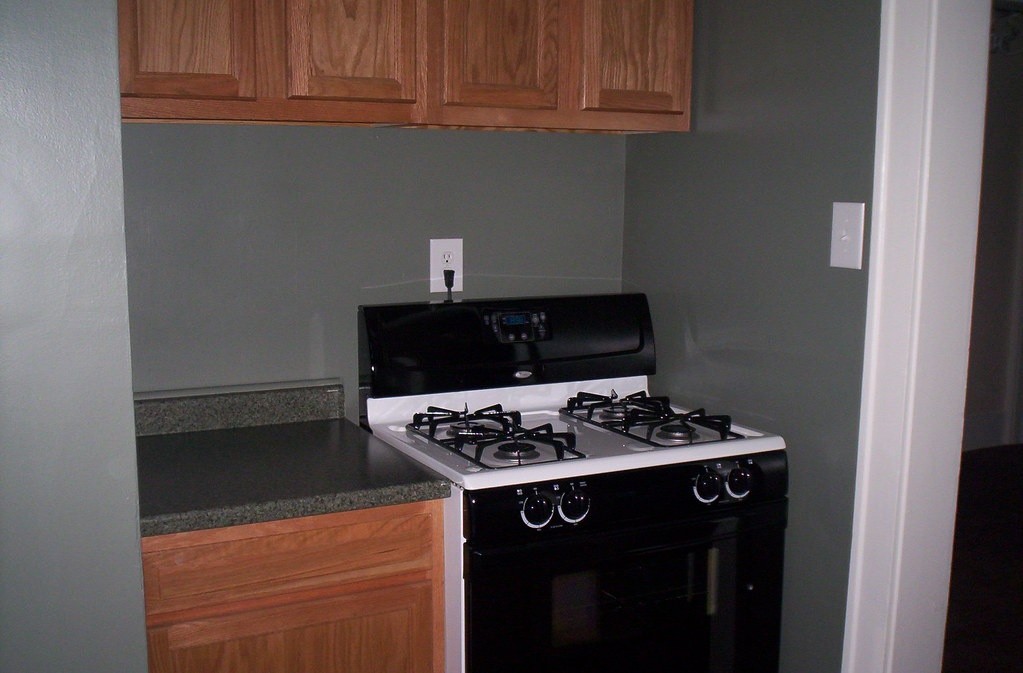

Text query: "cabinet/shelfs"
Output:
(115, 0), (692, 133)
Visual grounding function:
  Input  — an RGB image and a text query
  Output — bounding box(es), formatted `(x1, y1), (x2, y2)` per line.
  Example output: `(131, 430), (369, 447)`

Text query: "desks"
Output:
(140, 497), (446, 673)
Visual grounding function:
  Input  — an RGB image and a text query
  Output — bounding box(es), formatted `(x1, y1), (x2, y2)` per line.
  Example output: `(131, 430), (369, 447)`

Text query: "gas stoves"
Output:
(358, 295), (789, 492)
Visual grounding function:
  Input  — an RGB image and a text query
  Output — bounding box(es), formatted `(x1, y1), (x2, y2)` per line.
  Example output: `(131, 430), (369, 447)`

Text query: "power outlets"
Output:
(430, 238), (464, 294)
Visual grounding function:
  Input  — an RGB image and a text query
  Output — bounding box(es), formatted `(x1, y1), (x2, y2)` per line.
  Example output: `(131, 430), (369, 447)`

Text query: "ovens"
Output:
(443, 450), (789, 673)
